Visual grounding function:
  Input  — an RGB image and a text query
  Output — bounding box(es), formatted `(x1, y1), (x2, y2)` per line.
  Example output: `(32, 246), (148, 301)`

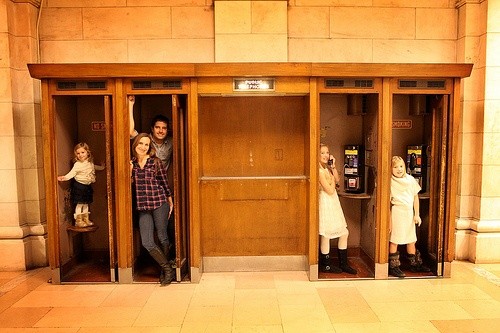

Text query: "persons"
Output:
(58, 143), (106, 228)
(128, 95), (174, 287)
(319, 143), (357, 275)
(389, 156), (431, 277)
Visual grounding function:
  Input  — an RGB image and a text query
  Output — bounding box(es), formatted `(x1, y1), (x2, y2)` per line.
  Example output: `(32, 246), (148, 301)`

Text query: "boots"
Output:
(337, 248), (357, 274)
(82, 212), (95, 226)
(389, 251), (406, 277)
(319, 253), (341, 273)
(160, 243), (172, 282)
(149, 245), (174, 285)
(74, 214), (88, 227)
(407, 249), (431, 272)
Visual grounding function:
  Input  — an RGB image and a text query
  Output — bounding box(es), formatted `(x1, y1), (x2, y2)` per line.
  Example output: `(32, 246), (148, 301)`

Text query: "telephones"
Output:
(328, 159), (333, 165)
(405, 144), (427, 194)
